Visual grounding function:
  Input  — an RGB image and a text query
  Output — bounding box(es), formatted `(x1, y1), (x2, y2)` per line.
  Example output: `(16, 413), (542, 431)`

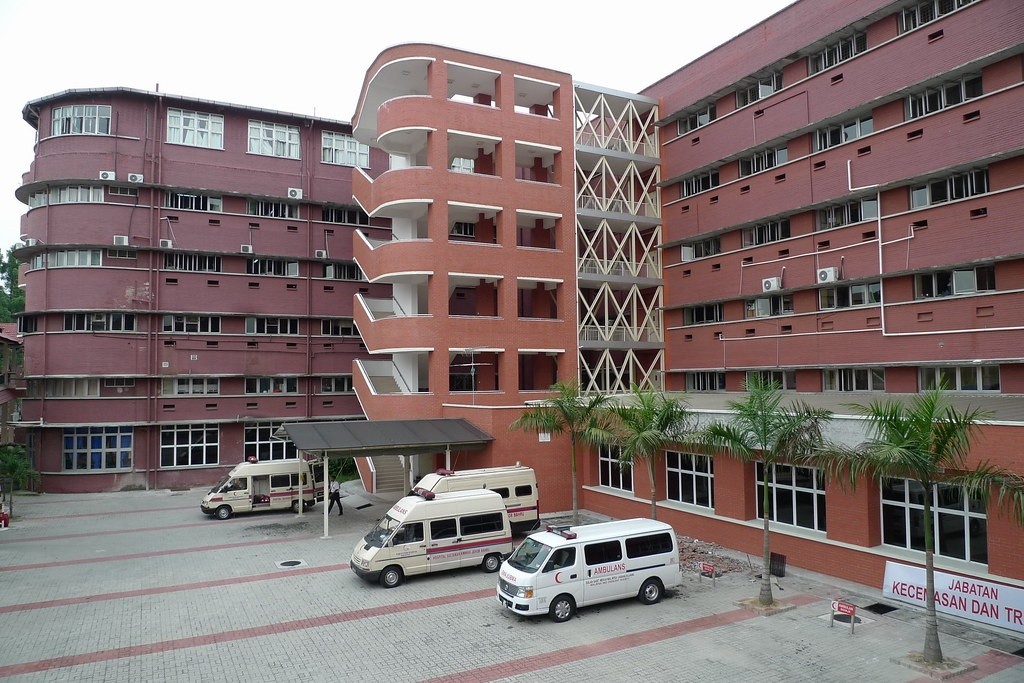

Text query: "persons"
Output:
(322, 476), (344, 516)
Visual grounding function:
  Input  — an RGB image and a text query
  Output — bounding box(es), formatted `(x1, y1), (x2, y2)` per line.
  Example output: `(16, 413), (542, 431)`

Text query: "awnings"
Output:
(269, 418), (496, 451)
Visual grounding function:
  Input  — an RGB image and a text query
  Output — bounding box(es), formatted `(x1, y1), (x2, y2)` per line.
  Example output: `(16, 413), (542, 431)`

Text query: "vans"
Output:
(496, 517), (683, 623)
(347, 487), (514, 588)
(200, 454), (331, 520)
(404, 462), (541, 537)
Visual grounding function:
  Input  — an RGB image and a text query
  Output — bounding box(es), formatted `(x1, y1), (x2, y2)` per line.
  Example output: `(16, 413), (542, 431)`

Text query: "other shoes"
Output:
(339, 513), (343, 516)
